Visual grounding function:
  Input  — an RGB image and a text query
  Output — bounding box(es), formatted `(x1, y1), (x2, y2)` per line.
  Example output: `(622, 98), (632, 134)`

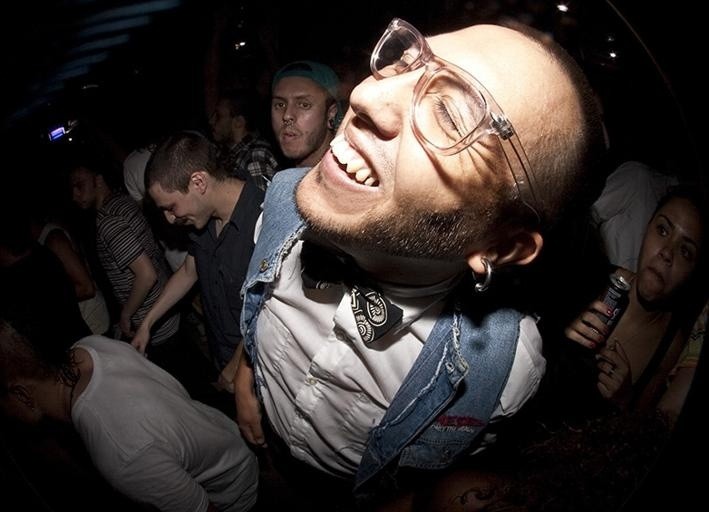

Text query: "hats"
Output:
(272, 60), (344, 131)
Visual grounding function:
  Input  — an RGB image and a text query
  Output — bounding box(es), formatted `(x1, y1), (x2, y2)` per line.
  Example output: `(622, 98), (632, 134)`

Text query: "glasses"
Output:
(369, 17), (545, 229)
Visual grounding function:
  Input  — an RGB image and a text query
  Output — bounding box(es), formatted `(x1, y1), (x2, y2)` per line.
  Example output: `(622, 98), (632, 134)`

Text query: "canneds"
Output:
(586, 274), (631, 348)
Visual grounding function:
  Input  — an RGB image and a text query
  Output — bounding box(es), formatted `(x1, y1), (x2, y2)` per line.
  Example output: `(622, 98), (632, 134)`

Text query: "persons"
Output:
(0, 305), (260, 510)
(500, 142), (708, 509)
(3, 61), (375, 430)
(234, 20), (608, 512)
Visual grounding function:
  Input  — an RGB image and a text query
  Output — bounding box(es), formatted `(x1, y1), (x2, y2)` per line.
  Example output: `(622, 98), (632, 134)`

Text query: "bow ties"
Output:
(300, 239), (403, 344)
